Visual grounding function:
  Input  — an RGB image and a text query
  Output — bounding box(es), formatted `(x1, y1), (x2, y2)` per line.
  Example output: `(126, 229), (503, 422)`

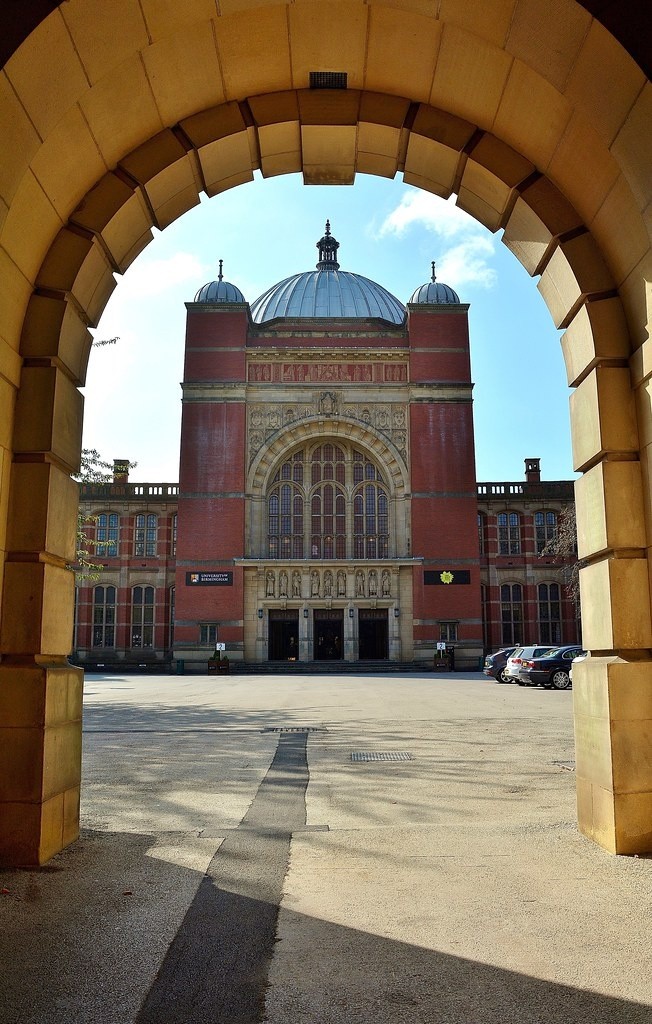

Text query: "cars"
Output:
(519, 645), (587, 690)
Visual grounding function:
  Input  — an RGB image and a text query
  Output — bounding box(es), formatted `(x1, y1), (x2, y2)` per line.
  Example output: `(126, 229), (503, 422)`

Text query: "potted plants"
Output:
(207, 655), (230, 675)
(434, 653), (452, 671)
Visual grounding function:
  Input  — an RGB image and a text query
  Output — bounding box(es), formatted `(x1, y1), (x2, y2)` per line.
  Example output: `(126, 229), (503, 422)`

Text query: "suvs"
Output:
(504, 645), (558, 686)
(482, 646), (520, 683)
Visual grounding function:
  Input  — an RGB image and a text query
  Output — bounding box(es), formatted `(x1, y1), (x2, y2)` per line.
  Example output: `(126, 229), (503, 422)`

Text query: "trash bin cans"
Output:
(479, 655), (485, 672)
(177, 659), (185, 675)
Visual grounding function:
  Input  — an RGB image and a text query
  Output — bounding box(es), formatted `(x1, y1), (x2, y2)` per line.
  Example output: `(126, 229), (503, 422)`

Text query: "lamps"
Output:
(259, 609), (264, 619)
(304, 609), (309, 619)
(349, 608), (355, 618)
(395, 608), (400, 617)
